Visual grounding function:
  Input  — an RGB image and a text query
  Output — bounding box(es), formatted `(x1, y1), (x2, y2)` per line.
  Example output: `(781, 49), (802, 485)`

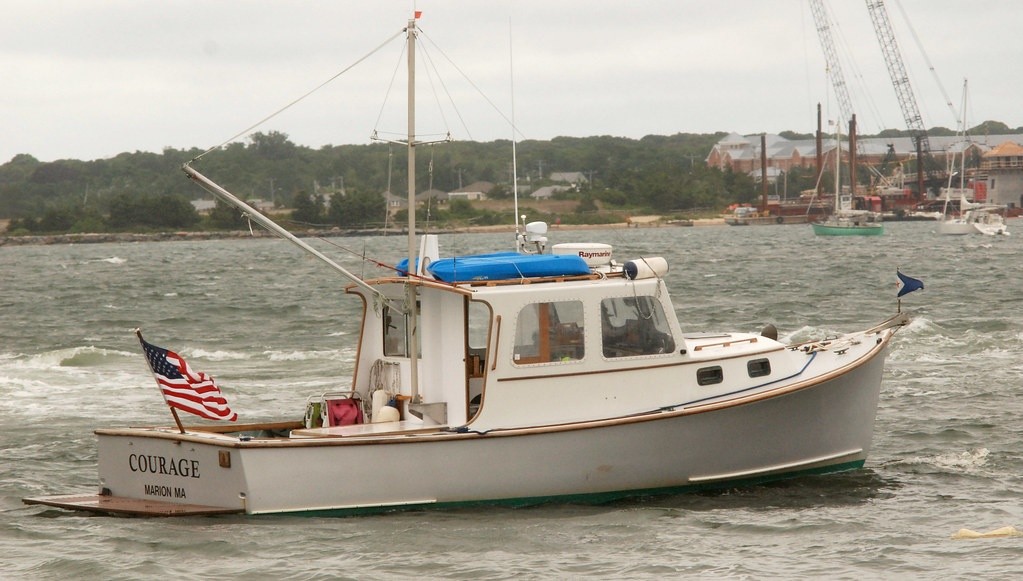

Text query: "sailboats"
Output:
(930, 79), (1007, 236)
(805, 115), (884, 236)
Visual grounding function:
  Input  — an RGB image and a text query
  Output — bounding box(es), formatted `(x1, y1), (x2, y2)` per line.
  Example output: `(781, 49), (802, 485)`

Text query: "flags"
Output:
(896, 271), (924, 298)
(142, 342), (237, 421)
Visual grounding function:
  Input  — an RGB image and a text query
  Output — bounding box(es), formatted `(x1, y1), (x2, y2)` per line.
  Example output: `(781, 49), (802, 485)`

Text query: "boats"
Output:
(20, 8), (909, 520)
(909, 192), (1006, 220)
(724, 211), (785, 226)
(666, 219), (695, 227)
